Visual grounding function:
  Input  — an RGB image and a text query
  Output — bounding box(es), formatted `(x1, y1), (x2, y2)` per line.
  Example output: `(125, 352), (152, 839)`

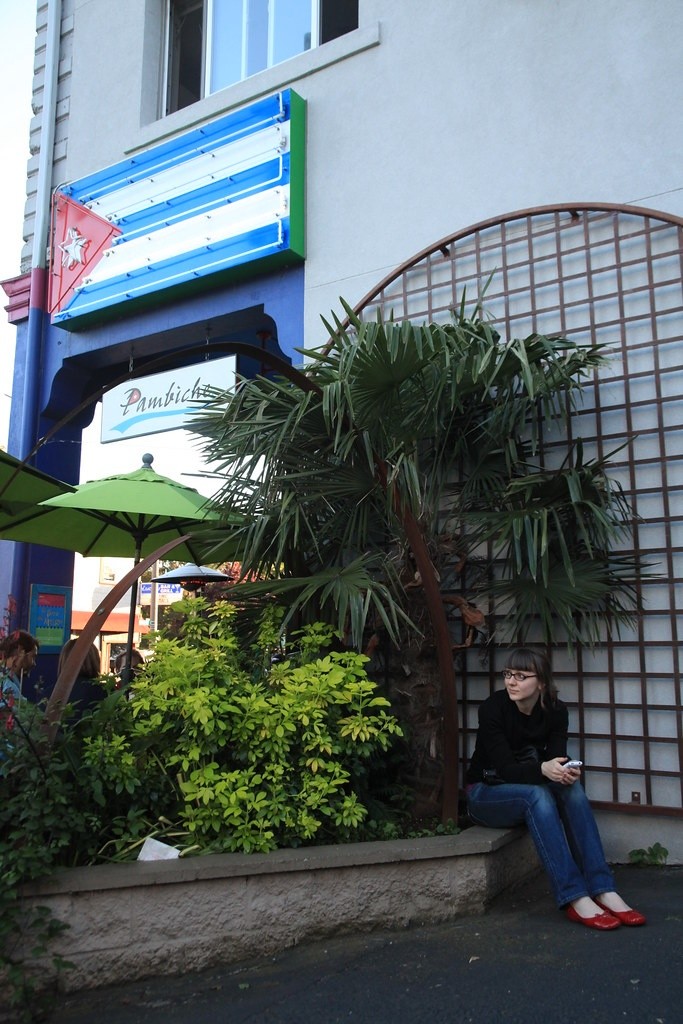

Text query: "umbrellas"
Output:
(1, 452), (256, 685)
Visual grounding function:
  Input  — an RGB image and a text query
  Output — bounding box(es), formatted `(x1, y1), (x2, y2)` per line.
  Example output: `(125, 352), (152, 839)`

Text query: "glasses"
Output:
(502, 669), (538, 681)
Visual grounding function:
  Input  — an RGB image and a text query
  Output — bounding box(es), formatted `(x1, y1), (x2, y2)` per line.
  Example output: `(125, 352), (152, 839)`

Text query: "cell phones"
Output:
(563, 760), (582, 769)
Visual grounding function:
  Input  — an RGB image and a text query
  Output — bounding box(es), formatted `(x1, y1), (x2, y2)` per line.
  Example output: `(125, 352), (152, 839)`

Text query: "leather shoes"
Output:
(593, 897), (647, 925)
(566, 900), (620, 930)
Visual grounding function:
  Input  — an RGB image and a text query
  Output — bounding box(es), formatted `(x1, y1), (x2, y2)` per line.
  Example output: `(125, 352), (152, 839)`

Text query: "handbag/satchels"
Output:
(480, 765), (551, 785)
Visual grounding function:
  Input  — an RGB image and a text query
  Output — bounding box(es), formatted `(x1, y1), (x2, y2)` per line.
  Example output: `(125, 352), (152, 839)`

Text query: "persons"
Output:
(464, 648), (645, 929)
(0, 628), (40, 771)
(115, 650), (144, 682)
(58, 638), (108, 727)
